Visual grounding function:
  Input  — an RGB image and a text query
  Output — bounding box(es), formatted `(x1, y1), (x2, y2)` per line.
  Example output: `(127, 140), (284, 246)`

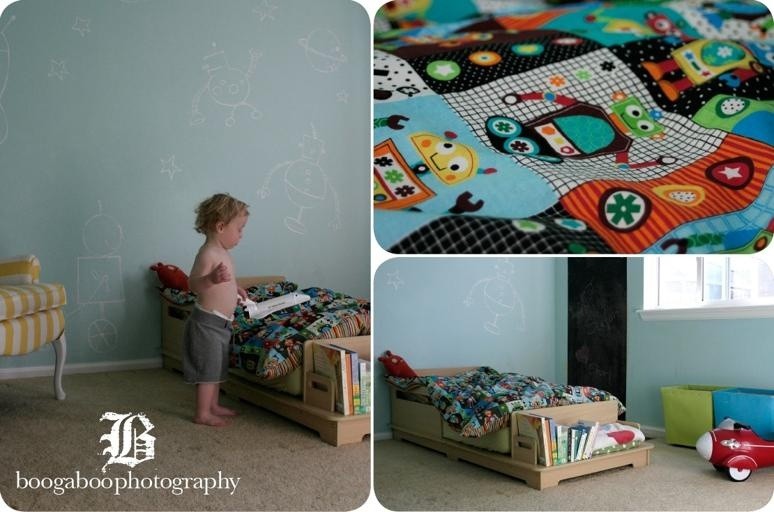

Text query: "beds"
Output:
(388, 366), (655, 491)
(159, 275), (370, 447)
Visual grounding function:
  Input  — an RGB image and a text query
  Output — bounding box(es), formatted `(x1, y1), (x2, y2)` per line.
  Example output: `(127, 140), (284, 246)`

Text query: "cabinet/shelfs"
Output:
(643, 257), (773, 320)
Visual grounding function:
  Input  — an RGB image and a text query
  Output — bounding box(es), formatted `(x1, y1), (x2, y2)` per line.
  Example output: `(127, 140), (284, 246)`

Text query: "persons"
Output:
(186, 191), (251, 427)
(695, 416), (773, 482)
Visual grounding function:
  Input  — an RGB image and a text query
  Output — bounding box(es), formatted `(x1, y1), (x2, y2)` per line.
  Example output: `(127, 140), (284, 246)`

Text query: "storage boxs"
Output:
(659, 383), (774, 449)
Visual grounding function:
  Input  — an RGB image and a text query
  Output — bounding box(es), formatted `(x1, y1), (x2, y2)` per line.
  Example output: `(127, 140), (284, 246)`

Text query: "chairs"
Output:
(0, 254), (70, 401)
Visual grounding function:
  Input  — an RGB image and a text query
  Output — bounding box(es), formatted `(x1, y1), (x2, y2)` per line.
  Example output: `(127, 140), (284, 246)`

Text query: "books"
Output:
(310, 341), (372, 416)
(513, 411), (601, 467)
